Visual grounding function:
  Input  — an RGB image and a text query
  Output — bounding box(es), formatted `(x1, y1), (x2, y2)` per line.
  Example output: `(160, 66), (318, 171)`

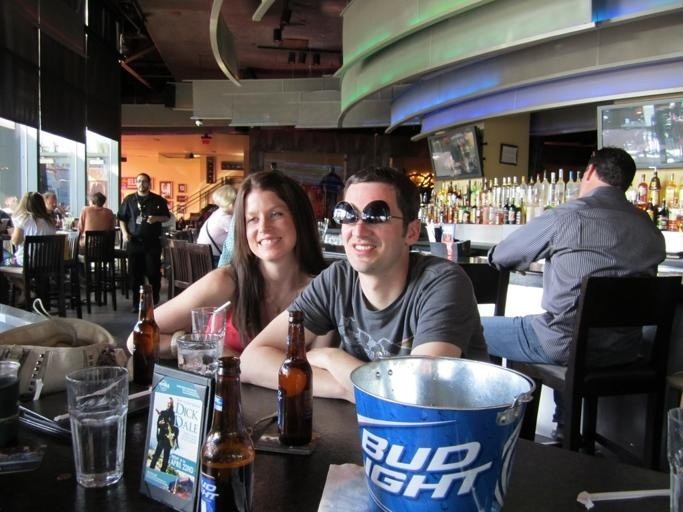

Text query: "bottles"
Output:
(276, 310), (312, 448)
(637, 174), (648, 202)
(643, 198), (656, 224)
(539, 170), (548, 207)
(554, 169), (564, 205)
(564, 170), (573, 203)
(573, 171), (580, 200)
(200, 357), (254, 512)
(667, 197), (679, 232)
(632, 191), (646, 211)
(663, 172), (675, 207)
(547, 172), (555, 206)
(677, 184), (682, 209)
(655, 198), (668, 231)
(648, 171), (660, 205)
(132, 284), (161, 388)
(419, 175), (541, 225)
(624, 182), (636, 205)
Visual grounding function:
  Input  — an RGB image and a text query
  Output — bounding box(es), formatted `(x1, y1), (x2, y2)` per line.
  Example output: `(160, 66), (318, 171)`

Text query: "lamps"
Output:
(273, 27), (280, 40)
(299, 52), (306, 63)
(312, 53), (320, 66)
(282, 10), (290, 24)
(287, 50), (296, 64)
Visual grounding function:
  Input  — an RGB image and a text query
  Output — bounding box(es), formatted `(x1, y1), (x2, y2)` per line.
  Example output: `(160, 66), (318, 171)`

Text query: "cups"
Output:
(64, 366), (129, 488)
(1, 358), (27, 451)
(176, 335), (222, 376)
(665, 405), (681, 510)
(190, 307), (227, 353)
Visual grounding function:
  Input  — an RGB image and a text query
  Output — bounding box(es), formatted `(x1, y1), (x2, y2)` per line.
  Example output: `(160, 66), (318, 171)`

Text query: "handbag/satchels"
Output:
(149, 221), (167, 248)
(0, 320), (126, 400)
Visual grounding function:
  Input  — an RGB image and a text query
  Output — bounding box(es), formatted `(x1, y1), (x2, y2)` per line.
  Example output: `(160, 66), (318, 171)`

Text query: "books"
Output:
(15, 379), (153, 445)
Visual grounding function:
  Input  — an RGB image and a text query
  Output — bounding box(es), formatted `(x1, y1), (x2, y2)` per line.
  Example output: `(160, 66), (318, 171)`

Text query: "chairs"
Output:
(506, 276), (682, 469)
(167, 239), (190, 300)
(113, 227), (134, 300)
(0, 235), (66, 317)
(189, 244), (213, 287)
(455, 263), (508, 364)
(67, 231), (117, 316)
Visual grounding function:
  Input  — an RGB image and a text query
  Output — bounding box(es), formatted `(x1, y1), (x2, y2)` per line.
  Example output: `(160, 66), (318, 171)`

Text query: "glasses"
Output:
(136, 179), (150, 182)
(332, 201), (407, 224)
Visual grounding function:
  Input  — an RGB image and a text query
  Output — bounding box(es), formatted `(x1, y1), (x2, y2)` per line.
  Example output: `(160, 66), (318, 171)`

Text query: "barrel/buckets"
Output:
(350, 354), (537, 512)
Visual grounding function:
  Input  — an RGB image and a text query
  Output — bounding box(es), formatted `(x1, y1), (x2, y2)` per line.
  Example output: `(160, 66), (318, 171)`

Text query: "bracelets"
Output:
(171, 330), (186, 359)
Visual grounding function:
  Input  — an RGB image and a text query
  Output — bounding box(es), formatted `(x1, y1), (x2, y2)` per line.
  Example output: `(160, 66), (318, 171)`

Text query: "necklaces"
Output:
(261, 282), (307, 318)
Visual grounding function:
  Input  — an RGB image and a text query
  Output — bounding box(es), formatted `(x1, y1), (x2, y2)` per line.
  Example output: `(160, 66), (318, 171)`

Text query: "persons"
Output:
(239, 166), (489, 405)
(42, 192), (69, 231)
(127, 169), (337, 364)
(195, 184), (237, 271)
(318, 162), (346, 226)
(117, 173), (171, 313)
(77, 191), (114, 255)
(0, 191), (56, 268)
(479, 147), (668, 366)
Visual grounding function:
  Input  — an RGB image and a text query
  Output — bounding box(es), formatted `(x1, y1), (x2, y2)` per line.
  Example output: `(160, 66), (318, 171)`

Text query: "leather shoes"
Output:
(132, 308), (139, 313)
